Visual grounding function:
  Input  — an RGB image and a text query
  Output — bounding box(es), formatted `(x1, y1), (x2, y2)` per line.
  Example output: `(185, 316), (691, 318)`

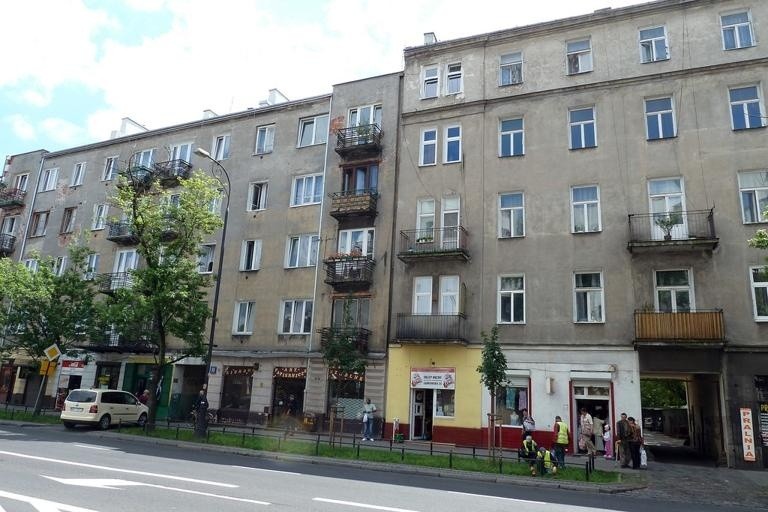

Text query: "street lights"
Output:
(193, 147), (231, 438)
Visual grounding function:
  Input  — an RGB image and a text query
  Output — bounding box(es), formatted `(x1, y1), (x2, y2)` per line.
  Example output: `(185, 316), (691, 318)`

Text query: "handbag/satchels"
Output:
(523, 421), (535, 431)
(581, 423), (592, 436)
(579, 436), (585, 450)
(640, 447), (648, 469)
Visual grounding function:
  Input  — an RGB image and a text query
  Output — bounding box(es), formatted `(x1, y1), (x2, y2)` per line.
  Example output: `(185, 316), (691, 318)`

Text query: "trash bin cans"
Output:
(303, 412), (318, 432)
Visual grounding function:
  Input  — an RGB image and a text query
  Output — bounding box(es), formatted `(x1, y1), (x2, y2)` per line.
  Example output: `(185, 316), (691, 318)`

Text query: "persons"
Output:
(195, 389), (209, 436)
(138, 389), (149, 405)
(359, 398), (378, 442)
(281, 393), (298, 440)
(515, 402), (642, 476)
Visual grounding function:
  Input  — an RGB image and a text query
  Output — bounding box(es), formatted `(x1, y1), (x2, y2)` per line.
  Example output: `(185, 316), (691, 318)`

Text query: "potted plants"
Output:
(653, 212), (680, 240)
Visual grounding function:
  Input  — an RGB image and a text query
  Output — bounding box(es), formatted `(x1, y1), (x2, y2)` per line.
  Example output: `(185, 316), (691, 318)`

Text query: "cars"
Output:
(61, 388), (152, 430)
